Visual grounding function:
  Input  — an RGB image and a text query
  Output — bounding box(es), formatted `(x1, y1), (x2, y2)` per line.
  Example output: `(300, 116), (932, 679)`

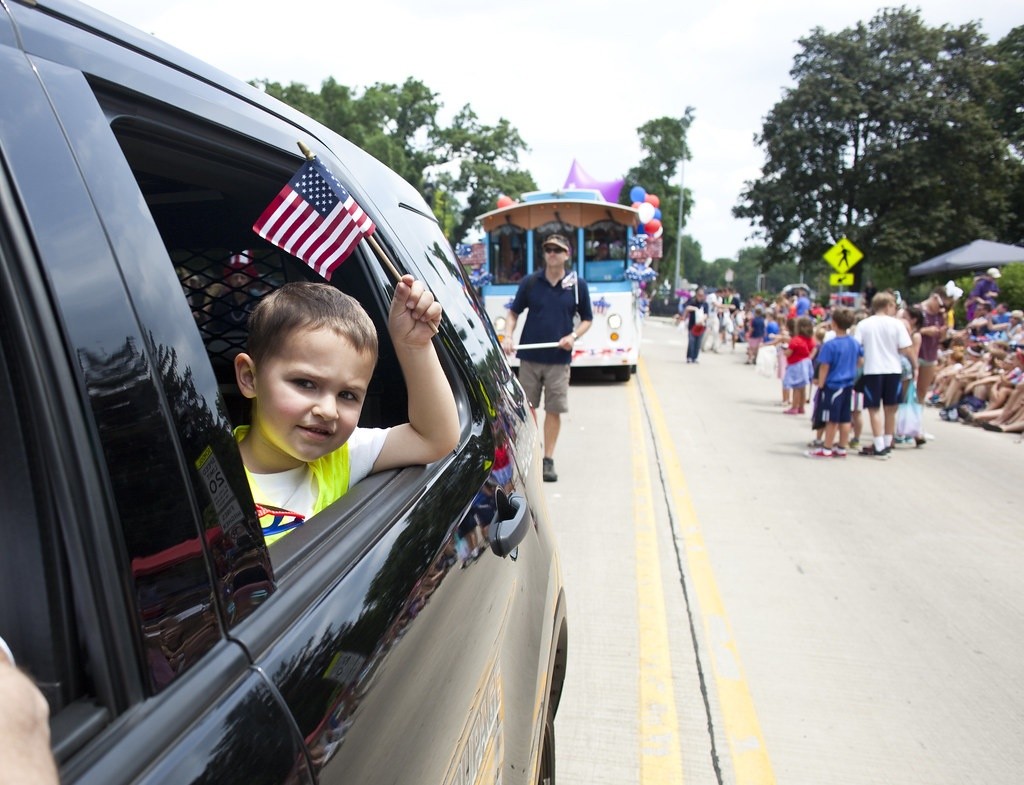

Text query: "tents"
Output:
(909, 238), (1024, 282)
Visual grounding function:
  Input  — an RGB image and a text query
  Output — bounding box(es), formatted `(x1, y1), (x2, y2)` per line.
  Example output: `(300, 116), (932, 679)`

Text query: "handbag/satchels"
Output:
(896, 380), (923, 437)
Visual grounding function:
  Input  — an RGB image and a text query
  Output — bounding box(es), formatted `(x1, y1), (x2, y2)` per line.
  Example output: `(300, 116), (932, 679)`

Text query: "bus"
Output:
(474, 187), (642, 383)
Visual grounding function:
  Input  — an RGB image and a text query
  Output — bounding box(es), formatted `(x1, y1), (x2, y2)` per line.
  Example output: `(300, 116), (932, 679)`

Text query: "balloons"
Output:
(630, 186), (699, 300)
(497, 196), (512, 207)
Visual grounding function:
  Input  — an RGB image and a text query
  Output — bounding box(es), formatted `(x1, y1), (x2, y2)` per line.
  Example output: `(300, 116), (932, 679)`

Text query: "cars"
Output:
(0, 0), (570, 785)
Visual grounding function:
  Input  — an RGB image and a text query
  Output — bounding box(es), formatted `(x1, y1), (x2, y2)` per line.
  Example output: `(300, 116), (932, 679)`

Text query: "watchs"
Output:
(571, 332), (578, 341)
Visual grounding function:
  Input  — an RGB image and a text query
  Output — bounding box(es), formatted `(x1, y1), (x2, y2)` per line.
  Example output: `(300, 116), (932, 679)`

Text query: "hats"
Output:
(542, 234), (570, 252)
(987, 268), (1002, 279)
(1012, 310), (1024, 320)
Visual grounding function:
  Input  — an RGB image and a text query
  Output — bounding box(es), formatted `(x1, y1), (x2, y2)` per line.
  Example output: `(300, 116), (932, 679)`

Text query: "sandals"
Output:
(808, 440), (822, 447)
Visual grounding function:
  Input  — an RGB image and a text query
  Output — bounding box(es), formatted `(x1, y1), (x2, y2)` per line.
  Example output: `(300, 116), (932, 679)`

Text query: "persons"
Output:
(177, 250), (274, 364)
(232, 275), (460, 548)
(291, 374), (526, 785)
(499, 234), (593, 482)
(638, 268), (1024, 460)
(0, 635), (62, 784)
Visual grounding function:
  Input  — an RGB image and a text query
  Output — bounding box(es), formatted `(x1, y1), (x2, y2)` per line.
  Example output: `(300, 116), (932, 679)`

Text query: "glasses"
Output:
(545, 247), (563, 253)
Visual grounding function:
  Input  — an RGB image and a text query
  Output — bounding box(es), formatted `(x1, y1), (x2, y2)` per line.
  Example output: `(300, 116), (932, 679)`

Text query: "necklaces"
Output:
(229, 301), (248, 321)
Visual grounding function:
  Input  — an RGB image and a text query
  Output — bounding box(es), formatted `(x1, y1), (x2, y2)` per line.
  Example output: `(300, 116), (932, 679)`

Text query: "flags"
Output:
(252, 154), (376, 281)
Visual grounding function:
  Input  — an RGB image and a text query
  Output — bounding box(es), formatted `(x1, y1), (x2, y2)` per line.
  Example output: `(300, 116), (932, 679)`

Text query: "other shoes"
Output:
(784, 407), (805, 414)
(895, 436), (904, 443)
(905, 437), (913, 443)
(849, 441), (860, 448)
(916, 438), (926, 445)
(858, 444), (892, 459)
(542, 458), (557, 481)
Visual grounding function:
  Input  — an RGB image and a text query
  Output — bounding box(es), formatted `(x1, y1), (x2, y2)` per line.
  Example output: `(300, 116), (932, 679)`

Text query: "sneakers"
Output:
(831, 446), (847, 457)
(805, 448), (833, 458)
